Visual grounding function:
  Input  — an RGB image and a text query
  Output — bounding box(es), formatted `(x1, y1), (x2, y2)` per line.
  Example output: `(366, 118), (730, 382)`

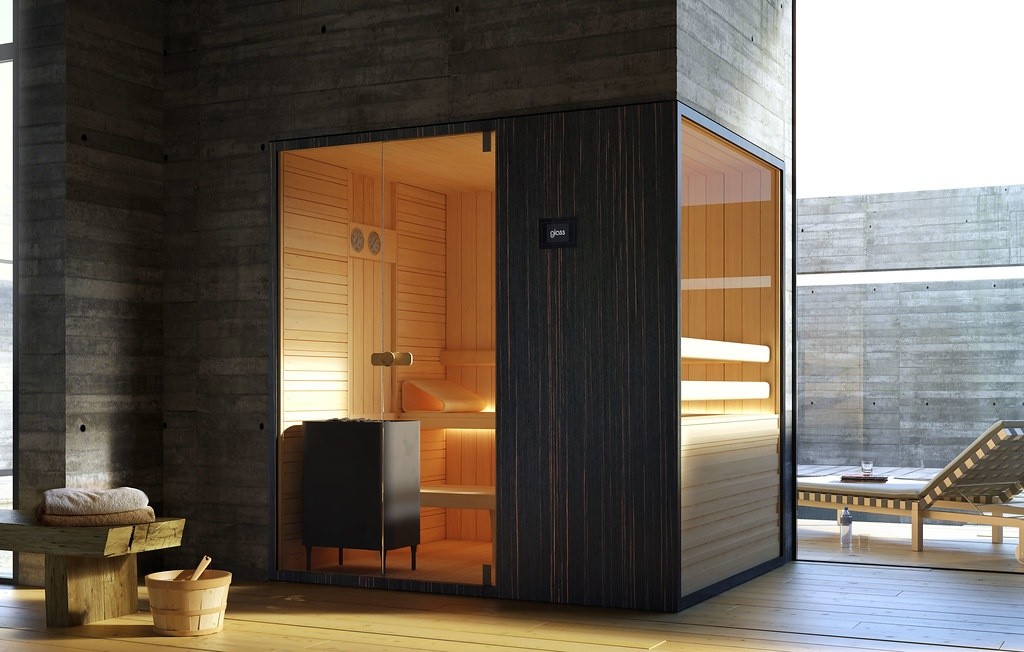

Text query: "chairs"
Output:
(796, 419), (1024, 564)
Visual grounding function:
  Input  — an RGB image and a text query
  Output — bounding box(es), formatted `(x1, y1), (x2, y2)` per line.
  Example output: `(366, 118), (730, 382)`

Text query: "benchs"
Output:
(0, 508), (186, 630)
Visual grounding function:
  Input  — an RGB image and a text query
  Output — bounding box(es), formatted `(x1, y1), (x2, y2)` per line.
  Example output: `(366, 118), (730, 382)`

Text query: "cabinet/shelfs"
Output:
(680, 337), (771, 402)
(397, 411), (495, 511)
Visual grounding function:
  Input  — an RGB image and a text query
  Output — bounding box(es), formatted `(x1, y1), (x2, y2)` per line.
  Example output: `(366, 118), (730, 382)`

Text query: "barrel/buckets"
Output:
(145, 569), (232, 637)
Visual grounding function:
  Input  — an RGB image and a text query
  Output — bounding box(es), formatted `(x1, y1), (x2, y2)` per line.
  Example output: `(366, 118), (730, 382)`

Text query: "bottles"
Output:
(840, 506), (852, 548)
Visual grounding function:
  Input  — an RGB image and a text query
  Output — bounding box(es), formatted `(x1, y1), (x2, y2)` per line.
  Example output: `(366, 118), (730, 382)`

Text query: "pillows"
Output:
(401, 377), (486, 413)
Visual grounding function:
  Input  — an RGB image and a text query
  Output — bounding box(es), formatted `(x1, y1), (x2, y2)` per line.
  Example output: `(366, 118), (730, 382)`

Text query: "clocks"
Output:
(349, 221), (397, 263)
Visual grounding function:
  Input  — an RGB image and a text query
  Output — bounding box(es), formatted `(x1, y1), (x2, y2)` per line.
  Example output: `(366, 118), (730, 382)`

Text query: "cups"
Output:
(861, 460), (873, 476)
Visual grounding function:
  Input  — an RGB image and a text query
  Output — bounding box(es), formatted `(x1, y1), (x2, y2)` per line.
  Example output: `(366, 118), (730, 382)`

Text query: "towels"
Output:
(37, 487), (155, 527)
